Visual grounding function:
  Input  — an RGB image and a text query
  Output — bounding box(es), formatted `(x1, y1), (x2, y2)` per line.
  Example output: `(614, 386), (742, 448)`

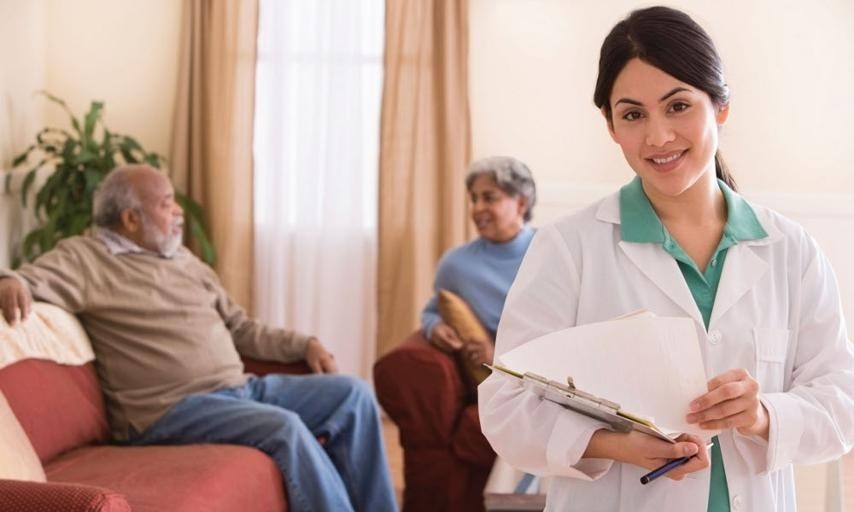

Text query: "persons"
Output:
(476, 6), (854, 512)
(421, 155), (537, 388)
(0, 164), (399, 512)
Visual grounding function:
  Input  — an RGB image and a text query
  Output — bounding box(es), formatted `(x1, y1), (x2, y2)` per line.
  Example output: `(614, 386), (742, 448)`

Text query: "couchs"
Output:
(0, 300), (330, 511)
(371, 327), (498, 511)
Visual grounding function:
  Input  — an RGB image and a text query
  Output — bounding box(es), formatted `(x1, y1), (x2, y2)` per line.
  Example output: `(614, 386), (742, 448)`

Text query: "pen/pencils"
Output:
(640, 442), (715, 485)
